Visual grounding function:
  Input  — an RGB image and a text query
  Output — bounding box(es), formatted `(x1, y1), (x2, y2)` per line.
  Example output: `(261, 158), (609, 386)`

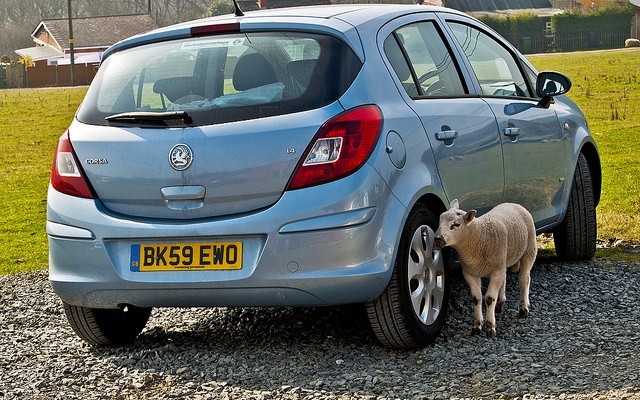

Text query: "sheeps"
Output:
(434, 199), (538, 338)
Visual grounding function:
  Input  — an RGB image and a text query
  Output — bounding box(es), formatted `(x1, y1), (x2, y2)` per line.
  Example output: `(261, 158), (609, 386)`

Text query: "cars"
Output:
(46, 4), (601, 349)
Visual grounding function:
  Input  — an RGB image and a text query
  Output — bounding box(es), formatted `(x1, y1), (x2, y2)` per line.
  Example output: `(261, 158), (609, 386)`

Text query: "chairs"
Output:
(232, 51), (280, 92)
(152, 75), (205, 108)
(381, 34), (428, 98)
(279, 59), (323, 99)
